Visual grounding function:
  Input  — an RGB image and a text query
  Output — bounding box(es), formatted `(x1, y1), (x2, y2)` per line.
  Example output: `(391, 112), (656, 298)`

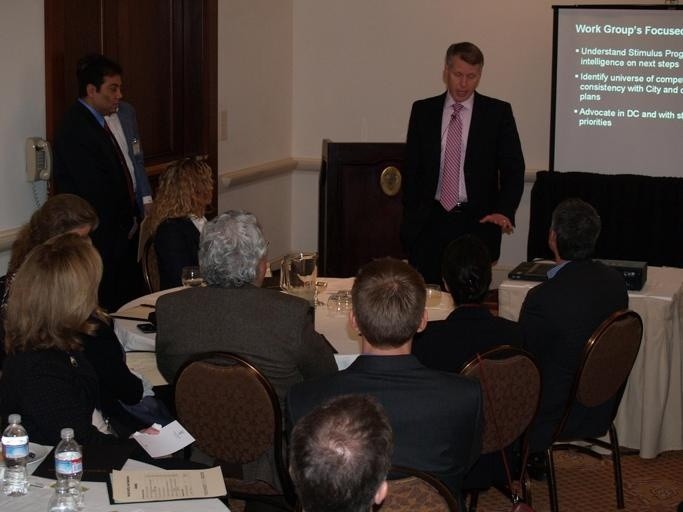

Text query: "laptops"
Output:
(506, 261), (555, 282)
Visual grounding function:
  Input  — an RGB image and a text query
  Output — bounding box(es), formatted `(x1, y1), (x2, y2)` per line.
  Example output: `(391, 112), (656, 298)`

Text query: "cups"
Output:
(315, 281), (327, 307)
(47, 492), (80, 512)
(326, 287), (353, 313)
(180, 265), (205, 288)
(424, 283), (440, 307)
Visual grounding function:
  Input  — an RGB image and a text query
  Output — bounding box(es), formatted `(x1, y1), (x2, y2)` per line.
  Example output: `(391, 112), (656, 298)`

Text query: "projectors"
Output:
(594, 260), (647, 291)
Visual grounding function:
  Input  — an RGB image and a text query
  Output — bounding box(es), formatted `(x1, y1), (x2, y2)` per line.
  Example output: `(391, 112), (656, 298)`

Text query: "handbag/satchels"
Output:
(476, 346), (534, 511)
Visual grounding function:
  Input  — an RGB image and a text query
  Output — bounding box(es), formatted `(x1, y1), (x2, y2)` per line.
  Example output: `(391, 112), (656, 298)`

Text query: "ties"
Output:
(440, 102), (463, 211)
(105, 120), (136, 208)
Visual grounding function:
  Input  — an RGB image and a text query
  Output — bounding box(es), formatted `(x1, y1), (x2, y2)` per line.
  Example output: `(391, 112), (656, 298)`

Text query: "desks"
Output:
(497, 263), (681, 462)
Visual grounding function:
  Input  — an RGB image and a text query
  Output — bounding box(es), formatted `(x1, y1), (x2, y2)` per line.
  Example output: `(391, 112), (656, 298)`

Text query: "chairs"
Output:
(142, 236), (164, 292)
(457, 343), (549, 512)
(373, 464), (458, 511)
(173, 350), (299, 512)
(529, 308), (648, 512)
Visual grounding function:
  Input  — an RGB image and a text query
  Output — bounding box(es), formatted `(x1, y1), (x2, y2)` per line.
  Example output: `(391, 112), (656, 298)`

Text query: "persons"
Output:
(0, 233), (143, 480)
(156, 212), (338, 432)
(144, 158), (215, 289)
(516, 200), (628, 481)
(288, 394), (392, 512)
(399, 42), (525, 283)
(53, 53), (152, 308)
(1, 193), (98, 312)
(415, 234), (527, 491)
(107, 96), (154, 204)
(287, 256), (481, 484)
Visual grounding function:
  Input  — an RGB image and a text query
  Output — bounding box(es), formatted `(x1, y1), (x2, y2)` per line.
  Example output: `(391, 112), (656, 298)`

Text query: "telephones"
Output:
(26, 137), (55, 183)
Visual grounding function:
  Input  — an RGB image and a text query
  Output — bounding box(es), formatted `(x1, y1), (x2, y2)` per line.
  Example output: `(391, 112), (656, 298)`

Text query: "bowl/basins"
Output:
(1, 440), (54, 483)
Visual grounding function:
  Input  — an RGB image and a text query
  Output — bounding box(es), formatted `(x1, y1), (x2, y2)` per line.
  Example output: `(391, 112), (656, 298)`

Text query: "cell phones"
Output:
(136, 323), (156, 334)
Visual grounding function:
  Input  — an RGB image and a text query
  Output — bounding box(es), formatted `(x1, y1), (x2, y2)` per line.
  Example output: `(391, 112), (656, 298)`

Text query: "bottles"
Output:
(2, 415), (30, 497)
(55, 429), (86, 509)
